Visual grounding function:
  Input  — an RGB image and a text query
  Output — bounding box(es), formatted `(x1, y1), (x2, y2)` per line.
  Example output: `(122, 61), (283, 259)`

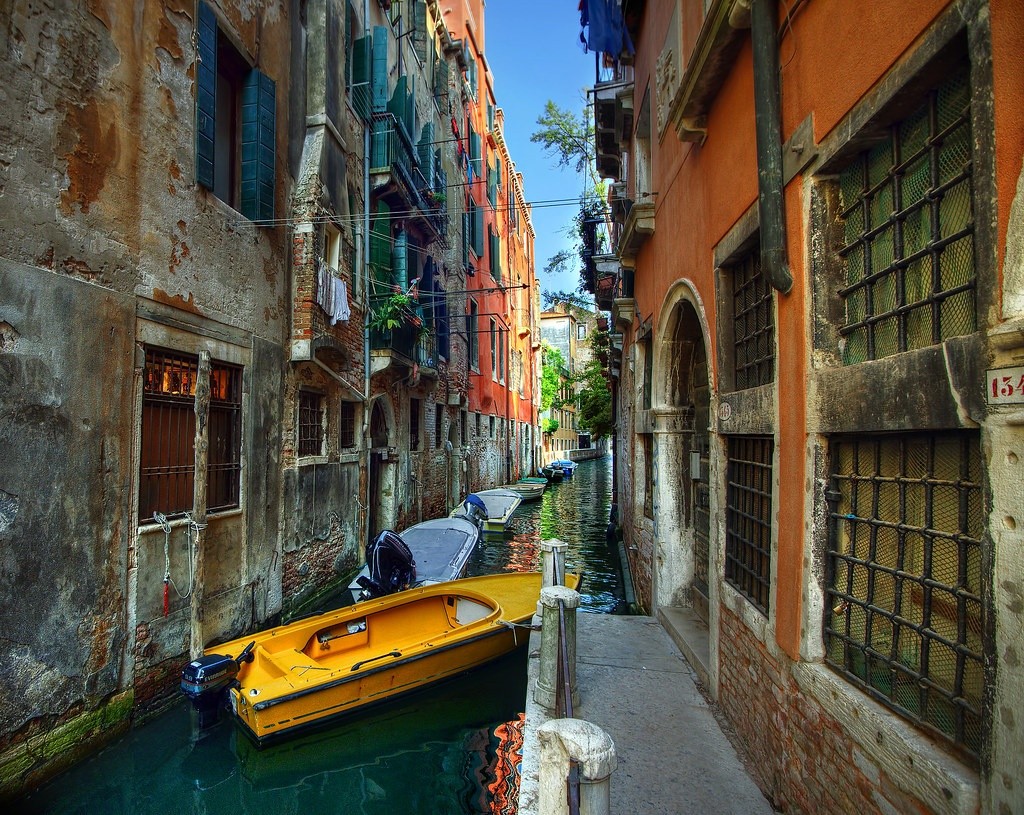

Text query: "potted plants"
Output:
(429, 191), (447, 209)
(593, 332), (609, 365)
(596, 271), (616, 291)
(365, 293), (408, 340)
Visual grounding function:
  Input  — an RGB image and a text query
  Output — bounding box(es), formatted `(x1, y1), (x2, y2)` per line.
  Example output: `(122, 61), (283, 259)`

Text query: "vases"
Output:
(596, 318), (608, 328)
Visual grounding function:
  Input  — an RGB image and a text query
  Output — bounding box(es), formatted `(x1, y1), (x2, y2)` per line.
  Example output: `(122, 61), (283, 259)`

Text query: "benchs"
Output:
(258, 647), (326, 677)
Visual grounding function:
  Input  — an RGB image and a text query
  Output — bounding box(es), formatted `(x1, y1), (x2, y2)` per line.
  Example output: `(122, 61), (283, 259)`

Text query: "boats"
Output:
(551, 459), (579, 477)
(348, 516), (479, 604)
(537, 465), (564, 482)
(503, 482), (547, 501)
(515, 475), (549, 488)
(226, 648), (528, 780)
(180, 569), (585, 750)
(447, 488), (524, 534)
